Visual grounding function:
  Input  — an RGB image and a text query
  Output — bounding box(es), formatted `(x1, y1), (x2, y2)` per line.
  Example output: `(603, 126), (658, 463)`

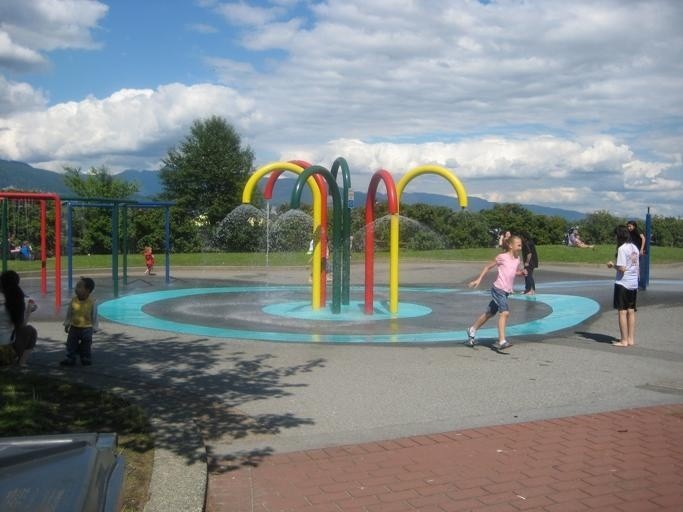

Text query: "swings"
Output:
(19, 202), (34, 256)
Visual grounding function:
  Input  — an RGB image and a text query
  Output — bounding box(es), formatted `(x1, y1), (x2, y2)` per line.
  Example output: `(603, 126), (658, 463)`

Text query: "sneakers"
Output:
(466, 327), (475, 346)
(490, 340), (512, 350)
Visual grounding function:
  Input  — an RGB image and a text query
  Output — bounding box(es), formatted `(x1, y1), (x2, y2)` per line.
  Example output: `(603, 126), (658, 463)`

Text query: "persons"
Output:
(59, 277), (98, 366)
(305, 239), (331, 282)
(0, 269), (38, 375)
(606, 224), (639, 346)
(5, 231), (36, 262)
(568, 229), (594, 249)
(462, 235), (528, 350)
(497, 230), (511, 254)
(143, 241), (154, 275)
(627, 221), (646, 256)
(518, 231), (539, 296)
